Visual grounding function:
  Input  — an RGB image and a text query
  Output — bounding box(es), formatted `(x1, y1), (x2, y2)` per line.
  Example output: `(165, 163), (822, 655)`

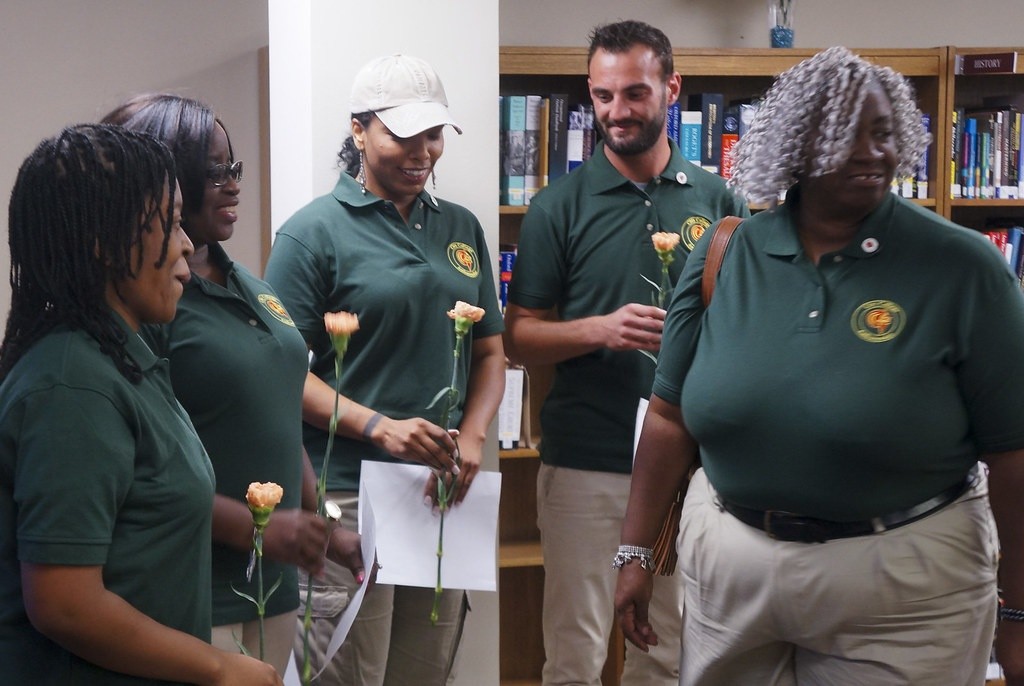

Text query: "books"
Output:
(497, 90), (1022, 444)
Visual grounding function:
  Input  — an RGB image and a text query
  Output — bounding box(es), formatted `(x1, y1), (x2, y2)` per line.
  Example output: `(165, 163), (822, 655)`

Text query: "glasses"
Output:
(205, 160), (243, 186)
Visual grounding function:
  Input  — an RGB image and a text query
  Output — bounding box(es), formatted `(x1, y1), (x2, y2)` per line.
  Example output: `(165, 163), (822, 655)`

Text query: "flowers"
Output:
(426, 300), (487, 620)
(299, 310), (360, 682)
(231, 481), (283, 661)
(634, 231), (681, 368)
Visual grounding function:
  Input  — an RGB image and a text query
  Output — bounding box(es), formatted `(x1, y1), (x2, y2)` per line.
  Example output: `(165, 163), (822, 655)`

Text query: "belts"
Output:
(733, 461), (983, 545)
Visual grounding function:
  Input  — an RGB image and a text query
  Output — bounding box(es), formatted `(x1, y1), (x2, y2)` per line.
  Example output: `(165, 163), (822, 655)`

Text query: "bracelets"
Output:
(999, 607), (1024, 623)
(610, 545), (657, 573)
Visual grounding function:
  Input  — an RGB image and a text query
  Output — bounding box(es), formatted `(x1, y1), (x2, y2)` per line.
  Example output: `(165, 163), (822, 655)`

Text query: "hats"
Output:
(350, 53), (463, 138)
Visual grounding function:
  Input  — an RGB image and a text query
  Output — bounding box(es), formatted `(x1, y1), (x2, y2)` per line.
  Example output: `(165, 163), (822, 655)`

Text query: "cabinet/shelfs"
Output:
(945, 46), (1024, 292)
(499, 45), (949, 685)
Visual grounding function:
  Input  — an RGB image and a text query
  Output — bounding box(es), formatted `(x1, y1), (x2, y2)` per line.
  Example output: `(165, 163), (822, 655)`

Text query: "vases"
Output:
(768, 0), (796, 48)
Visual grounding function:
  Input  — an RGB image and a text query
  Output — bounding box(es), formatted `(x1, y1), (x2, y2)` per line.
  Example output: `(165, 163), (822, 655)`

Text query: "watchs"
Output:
(313, 500), (343, 524)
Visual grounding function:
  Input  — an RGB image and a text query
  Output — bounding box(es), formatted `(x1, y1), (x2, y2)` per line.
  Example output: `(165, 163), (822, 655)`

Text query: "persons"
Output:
(0, 122), (285, 686)
(620, 46), (1024, 685)
(499, 20), (757, 686)
(91, 92), (364, 686)
(259, 53), (507, 686)
